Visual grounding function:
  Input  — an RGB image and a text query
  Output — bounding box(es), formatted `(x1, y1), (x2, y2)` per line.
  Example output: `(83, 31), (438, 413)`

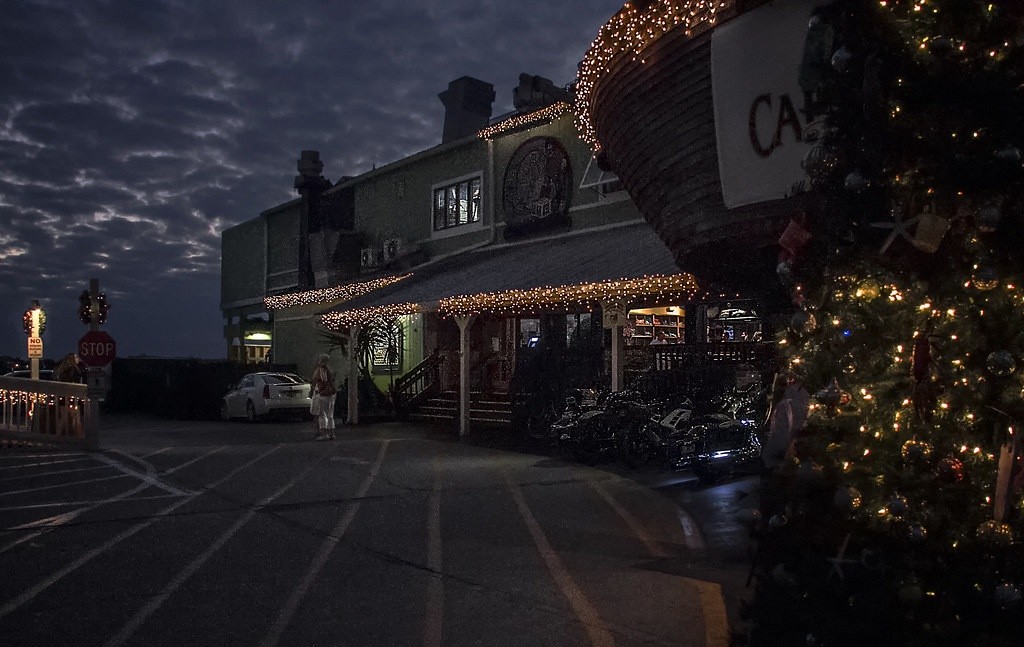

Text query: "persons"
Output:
(52, 353), (82, 436)
(308, 365), (336, 441)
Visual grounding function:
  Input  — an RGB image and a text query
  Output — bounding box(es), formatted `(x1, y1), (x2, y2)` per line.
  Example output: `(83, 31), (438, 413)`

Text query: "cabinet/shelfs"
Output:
(628, 313), (762, 346)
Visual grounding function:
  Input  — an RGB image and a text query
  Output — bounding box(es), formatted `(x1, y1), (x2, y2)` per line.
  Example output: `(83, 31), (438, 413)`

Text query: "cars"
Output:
(217, 372), (315, 424)
(3, 369), (55, 382)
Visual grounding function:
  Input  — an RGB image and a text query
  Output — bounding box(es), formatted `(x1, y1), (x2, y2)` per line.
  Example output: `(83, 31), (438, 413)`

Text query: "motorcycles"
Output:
(521, 363), (765, 480)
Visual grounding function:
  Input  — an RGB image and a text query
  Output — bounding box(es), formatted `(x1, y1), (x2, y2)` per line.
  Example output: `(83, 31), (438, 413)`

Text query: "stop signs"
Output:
(77, 330), (116, 368)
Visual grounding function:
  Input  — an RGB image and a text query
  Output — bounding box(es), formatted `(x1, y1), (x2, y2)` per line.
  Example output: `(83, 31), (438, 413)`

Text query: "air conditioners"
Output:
(383, 236), (407, 259)
(360, 248), (379, 266)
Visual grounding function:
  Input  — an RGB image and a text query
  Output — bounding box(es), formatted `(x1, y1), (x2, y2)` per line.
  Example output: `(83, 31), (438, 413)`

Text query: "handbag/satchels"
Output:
(310, 384), (320, 415)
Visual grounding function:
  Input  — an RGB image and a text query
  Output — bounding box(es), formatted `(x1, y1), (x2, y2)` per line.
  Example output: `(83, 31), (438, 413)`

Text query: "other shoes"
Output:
(316, 434), (329, 441)
(328, 433), (336, 440)
